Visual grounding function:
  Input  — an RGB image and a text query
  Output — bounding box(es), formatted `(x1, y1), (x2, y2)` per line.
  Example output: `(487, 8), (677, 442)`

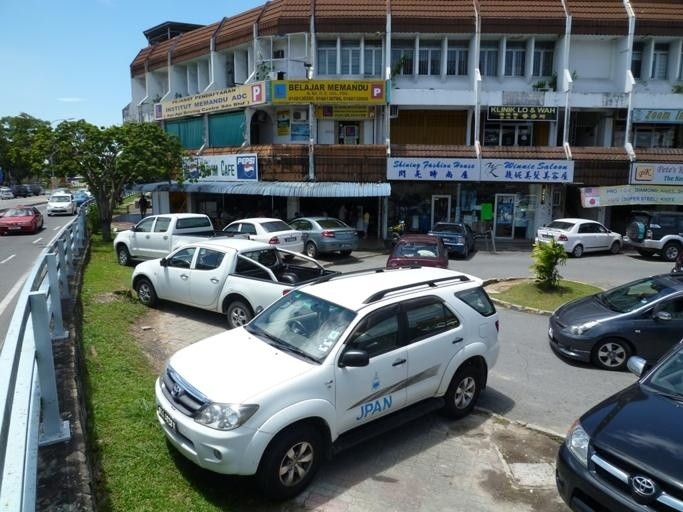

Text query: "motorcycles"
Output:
(387, 220), (406, 246)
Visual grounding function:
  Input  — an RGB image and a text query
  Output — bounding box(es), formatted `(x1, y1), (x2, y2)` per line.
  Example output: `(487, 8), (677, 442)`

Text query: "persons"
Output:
(361, 210), (369, 241)
(338, 204), (347, 223)
(294, 211), (300, 218)
(136, 195), (148, 219)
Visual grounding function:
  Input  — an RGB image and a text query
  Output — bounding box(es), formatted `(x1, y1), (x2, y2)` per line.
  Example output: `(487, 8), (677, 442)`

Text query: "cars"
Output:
(220, 217), (306, 255)
(0, 181), (93, 235)
(387, 232), (453, 273)
(428, 220), (478, 260)
(286, 216), (359, 261)
(546, 269), (683, 370)
(534, 217), (623, 257)
(554, 337), (683, 512)
(503, 193), (538, 230)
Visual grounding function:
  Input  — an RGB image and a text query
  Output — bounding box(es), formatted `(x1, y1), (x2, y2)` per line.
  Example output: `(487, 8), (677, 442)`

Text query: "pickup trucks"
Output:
(129, 238), (343, 330)
(112, 212), (250, 267)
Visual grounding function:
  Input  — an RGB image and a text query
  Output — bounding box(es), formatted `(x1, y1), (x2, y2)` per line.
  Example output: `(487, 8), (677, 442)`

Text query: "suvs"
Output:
(624, 209), (683, 262)
(154, 266), (503, 501)
